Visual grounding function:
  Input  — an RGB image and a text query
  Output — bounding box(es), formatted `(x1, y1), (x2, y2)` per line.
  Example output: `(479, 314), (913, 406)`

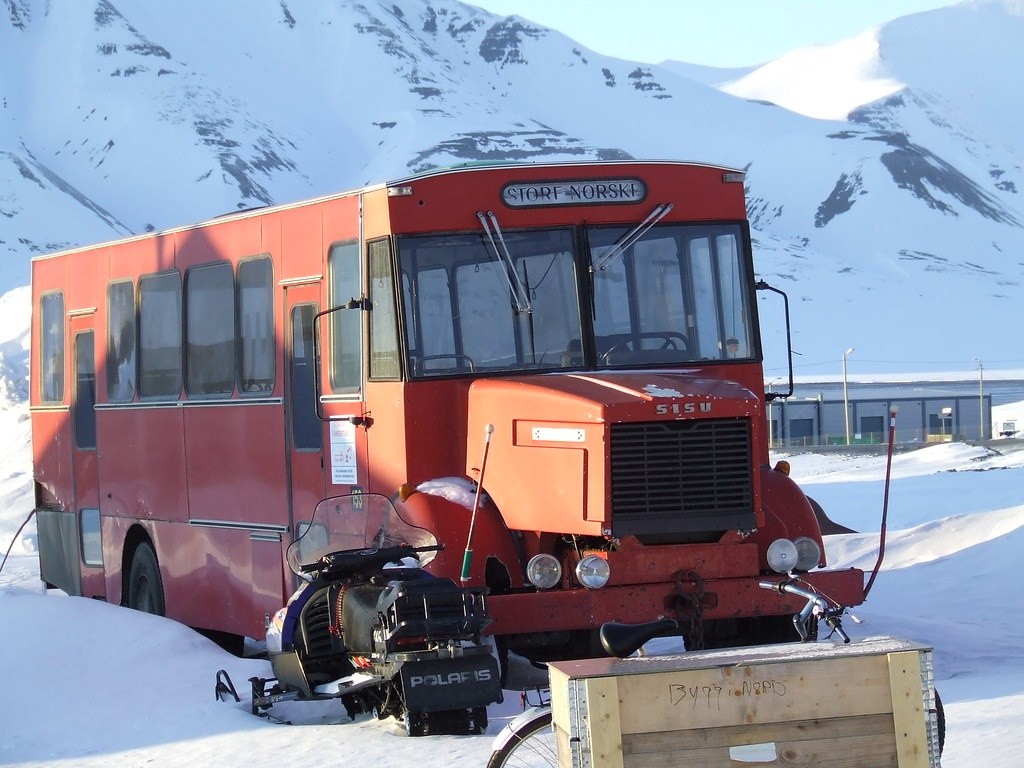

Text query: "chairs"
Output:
(415, 353), (479, 377)
(566, 335), (633, 367)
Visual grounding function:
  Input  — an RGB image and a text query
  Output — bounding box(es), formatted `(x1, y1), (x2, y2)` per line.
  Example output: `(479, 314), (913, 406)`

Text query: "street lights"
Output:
(768, 377), (783, 449)
(842, 348), (856, 446)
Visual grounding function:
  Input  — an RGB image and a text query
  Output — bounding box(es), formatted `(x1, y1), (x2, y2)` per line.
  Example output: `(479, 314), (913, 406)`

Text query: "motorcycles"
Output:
(214, 494), (503, 737)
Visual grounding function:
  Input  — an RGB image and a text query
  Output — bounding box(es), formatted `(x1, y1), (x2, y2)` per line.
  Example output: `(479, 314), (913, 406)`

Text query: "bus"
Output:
(25, 159), (900, 691)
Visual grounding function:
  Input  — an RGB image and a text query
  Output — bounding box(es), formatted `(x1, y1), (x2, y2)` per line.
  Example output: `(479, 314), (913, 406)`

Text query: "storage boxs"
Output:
(545, 632), (942, 768)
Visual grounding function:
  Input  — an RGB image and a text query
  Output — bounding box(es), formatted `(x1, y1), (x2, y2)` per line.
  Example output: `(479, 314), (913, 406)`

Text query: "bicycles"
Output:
(486, 578), (946, 767)
